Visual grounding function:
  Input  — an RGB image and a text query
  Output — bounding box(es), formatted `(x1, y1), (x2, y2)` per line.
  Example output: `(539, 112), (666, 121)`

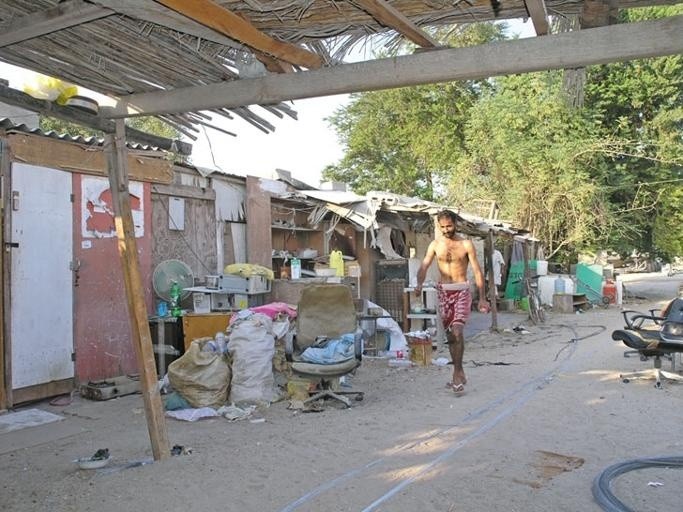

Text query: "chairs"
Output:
(611, 296), (682, 387)
(285, 282), (365, 408)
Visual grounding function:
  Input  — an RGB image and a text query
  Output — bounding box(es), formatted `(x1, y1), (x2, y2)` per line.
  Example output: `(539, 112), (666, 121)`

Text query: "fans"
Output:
(151, 259), (194, 304)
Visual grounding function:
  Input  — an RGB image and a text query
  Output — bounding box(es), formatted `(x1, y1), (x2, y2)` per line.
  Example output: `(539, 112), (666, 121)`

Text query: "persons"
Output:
(414, 209), (493, 397)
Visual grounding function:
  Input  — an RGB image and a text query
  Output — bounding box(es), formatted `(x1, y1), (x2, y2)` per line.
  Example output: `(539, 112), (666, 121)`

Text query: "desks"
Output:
(358, 315), (399, 357)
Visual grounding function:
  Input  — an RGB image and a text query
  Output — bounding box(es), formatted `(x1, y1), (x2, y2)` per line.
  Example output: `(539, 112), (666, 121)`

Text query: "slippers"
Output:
(445, 380), (464, 394)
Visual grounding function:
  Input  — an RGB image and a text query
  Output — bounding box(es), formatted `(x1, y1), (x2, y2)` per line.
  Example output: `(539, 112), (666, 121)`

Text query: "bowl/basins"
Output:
(68, 455), (114, 470)
(203, 275), (219, 289)
(315, 267), (336, 276)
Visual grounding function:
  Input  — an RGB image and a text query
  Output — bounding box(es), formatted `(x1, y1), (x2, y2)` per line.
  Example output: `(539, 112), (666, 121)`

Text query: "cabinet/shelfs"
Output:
(182, 311), (236, 354)
(551, 293), (587, 313)
(245, 189), (325, 281)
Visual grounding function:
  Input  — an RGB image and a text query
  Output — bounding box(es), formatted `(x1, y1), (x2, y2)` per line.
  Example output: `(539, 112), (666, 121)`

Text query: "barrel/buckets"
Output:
(329, 248), (344, 279)
(404, 332), (433, 365)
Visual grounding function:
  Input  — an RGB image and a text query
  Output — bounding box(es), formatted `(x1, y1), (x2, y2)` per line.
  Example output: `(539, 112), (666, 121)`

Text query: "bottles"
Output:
(169, 282), (182, 317)
(201, 339), (216, 353)
(156, 301), (168, 317)
(377, 350), (404, 358)
(215, 331), (227, 354)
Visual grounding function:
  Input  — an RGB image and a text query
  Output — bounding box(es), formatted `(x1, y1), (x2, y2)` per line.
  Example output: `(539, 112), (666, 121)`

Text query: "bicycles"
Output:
(510, 272), (547, 326)
